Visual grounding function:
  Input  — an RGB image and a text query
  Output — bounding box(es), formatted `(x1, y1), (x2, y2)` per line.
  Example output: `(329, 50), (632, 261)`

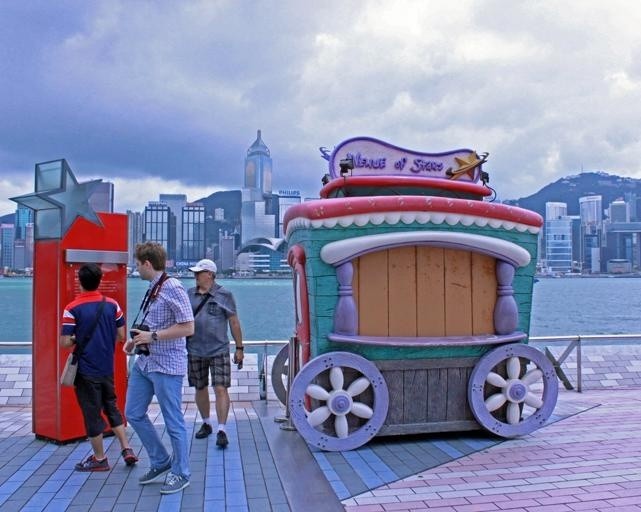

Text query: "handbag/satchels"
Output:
(61, 352), (78, 388)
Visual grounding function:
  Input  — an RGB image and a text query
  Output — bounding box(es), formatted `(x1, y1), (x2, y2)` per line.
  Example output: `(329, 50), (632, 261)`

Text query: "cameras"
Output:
(130, 324), (151, 356)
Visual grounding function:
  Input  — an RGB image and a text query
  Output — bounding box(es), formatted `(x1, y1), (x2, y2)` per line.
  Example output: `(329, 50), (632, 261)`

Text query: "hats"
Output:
(187, 259), (217, 275)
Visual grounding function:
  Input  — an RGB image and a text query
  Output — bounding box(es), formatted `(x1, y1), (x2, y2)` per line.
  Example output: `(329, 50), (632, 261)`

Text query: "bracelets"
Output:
(236, 347), (244, 351)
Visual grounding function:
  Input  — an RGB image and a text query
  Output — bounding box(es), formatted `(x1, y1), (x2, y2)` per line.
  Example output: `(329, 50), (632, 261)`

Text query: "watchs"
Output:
(152, 330), (157, 341)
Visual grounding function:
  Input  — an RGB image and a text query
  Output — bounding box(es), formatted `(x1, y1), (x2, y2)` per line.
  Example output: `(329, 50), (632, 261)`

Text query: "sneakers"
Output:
(194, 423), (212, 439)
(120, 448), (138, 465)
(160, 471), (190, 494)
(73, 454), (110, 472)
(215, 431), (228, 447)
(137, 455), (172, 485)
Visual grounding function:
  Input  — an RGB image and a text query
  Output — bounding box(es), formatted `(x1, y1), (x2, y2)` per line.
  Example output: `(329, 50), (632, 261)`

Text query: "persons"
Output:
(59, 262), (138, 471)
(122, 240), (195, 494)
(187, 259), (244, 446)
